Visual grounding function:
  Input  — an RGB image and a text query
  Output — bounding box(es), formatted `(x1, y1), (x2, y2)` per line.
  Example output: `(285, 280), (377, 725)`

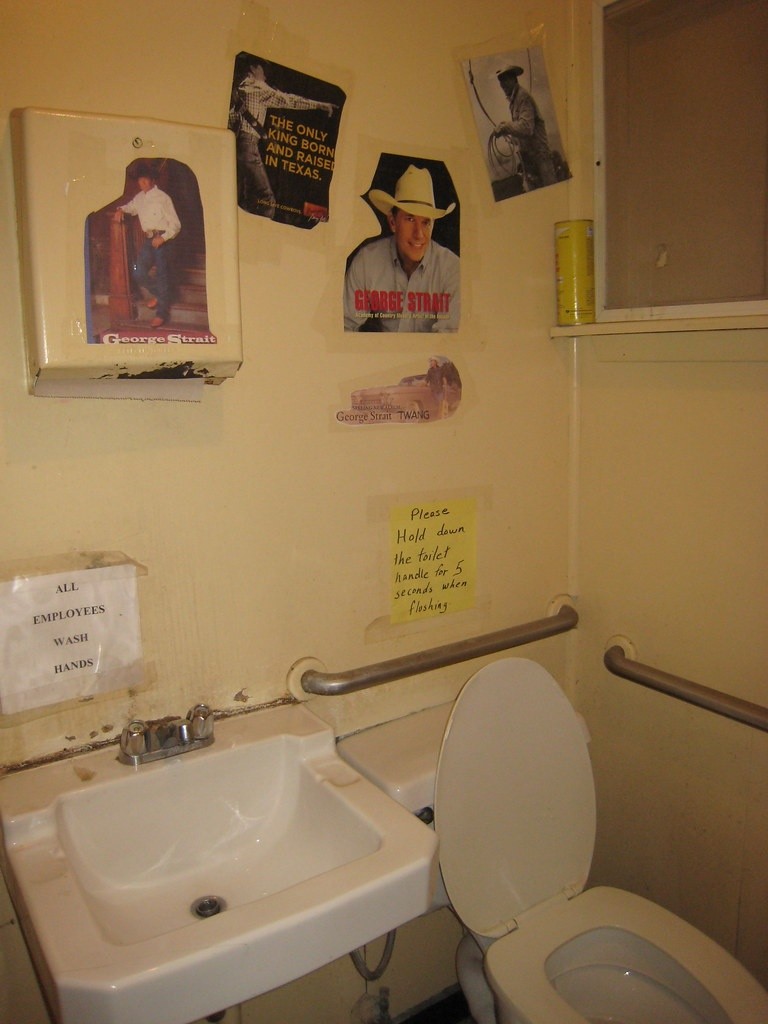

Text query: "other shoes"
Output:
(146, 296), (159, 309)
(149, 316), (166, 329)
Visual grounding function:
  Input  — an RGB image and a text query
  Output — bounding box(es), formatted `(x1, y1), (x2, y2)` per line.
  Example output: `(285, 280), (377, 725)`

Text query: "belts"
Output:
(228, 128), (260, 144)
(143, 229), (166, 240)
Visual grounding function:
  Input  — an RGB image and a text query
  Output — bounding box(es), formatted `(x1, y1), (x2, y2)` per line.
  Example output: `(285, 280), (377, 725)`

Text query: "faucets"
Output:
(117, 703), (213, 765)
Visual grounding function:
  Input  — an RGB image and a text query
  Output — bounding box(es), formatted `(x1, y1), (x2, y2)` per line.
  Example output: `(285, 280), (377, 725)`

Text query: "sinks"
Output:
(1, 700), (441, 1022)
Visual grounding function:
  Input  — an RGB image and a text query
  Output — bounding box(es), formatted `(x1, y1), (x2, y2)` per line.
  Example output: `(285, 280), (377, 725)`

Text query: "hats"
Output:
(368, 163), (456, 220)
(235, 53), (270, 72)
(495, 63), (525, 77)
(129, 163), (161, 180)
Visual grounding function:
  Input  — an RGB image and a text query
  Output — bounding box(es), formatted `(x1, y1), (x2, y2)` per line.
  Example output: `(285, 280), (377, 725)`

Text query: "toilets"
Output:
(435, 654), (767, 1024)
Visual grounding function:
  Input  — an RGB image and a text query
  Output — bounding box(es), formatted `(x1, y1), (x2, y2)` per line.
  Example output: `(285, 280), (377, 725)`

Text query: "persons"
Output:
(344, 164), (460, 332)
(227, 53), (340, 219)
(416, 356), (445, 420)
(496, 64), (558, 192)
(114, 163), (182, 328)
